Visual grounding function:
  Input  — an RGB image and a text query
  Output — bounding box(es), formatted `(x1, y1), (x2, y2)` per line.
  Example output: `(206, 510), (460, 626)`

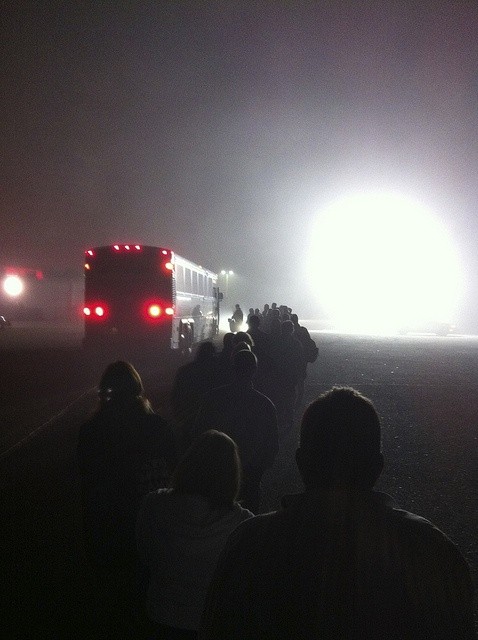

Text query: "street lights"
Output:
(220, 270), (233, 295)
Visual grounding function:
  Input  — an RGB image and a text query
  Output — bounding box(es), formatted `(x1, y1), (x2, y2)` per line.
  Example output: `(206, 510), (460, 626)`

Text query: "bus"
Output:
(82, 244), (223, 355)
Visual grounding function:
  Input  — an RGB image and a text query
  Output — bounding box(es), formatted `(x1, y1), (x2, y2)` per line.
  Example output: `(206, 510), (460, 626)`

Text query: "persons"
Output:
(279, 361), (317, 431)
(187, 351), (278, 515)
(169, 340), (220, 428)
(136, 431), (255, 639)
(76, 361), (177, 637)
(201, 385), (477, 634)
(228, 304), (243, 332)
(219, 343), (252, 394)
(232, 332), (255, 352)
(246, 304), (323, 360)
